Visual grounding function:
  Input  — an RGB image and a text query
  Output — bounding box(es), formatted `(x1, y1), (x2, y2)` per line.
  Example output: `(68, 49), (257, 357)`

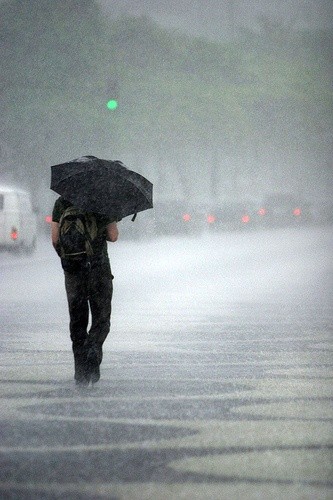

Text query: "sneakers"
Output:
(87, 348), (100, 382)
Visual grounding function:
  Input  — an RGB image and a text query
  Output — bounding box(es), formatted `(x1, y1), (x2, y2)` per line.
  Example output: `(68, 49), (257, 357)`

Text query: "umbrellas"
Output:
(49, 155), (155, 223)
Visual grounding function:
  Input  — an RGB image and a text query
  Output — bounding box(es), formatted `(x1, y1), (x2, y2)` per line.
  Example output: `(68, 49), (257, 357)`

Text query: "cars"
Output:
(148, 196), (324, 234)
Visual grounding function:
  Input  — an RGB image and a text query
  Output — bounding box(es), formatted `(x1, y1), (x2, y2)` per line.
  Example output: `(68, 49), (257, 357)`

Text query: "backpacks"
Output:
(58, 195), (104, 272)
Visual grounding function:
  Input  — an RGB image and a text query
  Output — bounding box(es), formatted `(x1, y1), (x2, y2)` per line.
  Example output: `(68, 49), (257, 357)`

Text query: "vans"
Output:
(0, 187), (38, 256)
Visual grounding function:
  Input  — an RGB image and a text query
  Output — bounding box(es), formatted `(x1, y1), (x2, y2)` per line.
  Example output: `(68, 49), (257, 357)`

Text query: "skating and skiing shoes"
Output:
(75, 375), (90, 386)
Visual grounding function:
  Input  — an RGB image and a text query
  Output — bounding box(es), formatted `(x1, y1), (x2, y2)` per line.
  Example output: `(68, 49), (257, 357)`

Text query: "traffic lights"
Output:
(107, 78), (119, 115)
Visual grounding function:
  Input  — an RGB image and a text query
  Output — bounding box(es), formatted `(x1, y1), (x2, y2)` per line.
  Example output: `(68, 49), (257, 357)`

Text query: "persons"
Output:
(47, 156), (118, 393)
